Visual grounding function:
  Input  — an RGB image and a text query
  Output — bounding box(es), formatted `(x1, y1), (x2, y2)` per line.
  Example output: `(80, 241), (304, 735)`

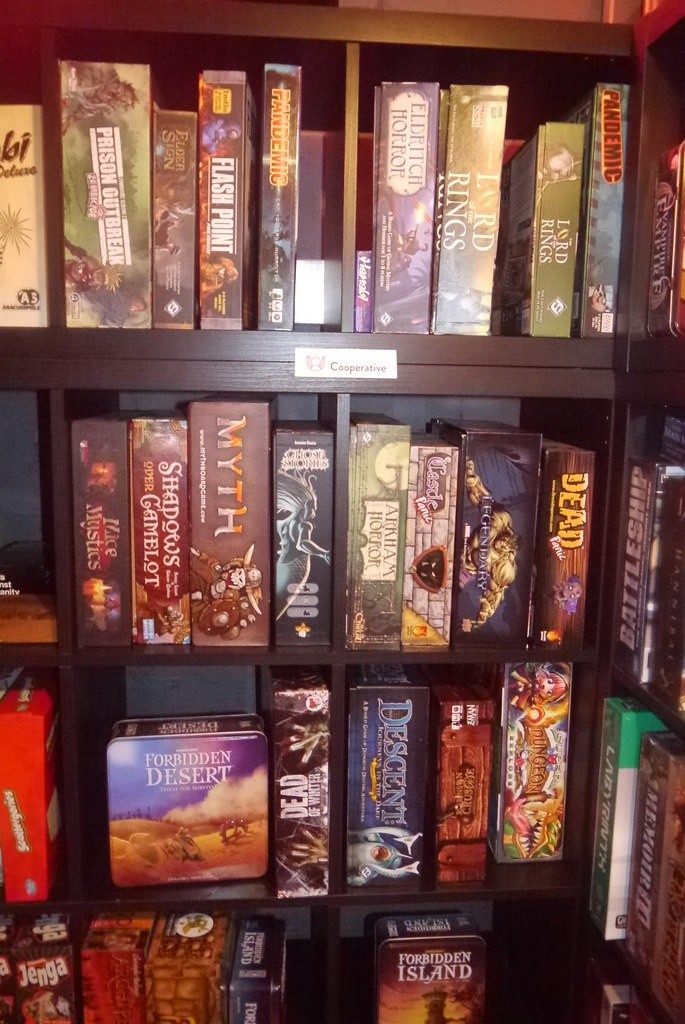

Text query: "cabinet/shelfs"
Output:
(585, 0), (685, 1024)
(0, 0), (632, 1024)
